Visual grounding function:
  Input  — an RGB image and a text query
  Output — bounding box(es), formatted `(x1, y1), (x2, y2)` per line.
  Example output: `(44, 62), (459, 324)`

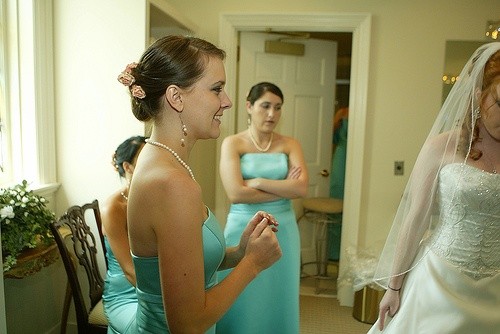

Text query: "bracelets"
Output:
(387, 285), (402, 292)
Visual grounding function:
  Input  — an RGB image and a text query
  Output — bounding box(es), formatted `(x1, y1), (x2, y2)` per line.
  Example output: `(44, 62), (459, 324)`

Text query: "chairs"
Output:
(49, 199), (108, 334)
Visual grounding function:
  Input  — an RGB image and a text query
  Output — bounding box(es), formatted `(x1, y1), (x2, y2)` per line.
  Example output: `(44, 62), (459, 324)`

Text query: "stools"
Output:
(296, 198), (343, 295)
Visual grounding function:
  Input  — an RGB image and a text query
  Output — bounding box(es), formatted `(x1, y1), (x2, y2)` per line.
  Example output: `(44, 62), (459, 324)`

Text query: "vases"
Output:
(2, 228), (77, 334)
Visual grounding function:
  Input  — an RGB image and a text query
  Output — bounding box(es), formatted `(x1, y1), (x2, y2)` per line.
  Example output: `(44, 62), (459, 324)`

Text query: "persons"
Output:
(119, 35), (283, 334)
(365, 41), (500, 334)
(100, 135), (151, 334)
(327, 108), (349, 260)
(214, 82), (308, 334)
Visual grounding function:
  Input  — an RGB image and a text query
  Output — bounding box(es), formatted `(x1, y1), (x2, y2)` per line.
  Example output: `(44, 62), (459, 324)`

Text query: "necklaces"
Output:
(479, 141), (498, 174)
(249, 128), (273, 152)
(144, 138), (196, 182)
(121, 191), (128, 201)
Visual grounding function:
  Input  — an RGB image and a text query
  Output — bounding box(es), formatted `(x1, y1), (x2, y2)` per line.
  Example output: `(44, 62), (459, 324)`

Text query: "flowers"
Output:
(0, 180), (58, 274)
(116, 62), (146, 100)
